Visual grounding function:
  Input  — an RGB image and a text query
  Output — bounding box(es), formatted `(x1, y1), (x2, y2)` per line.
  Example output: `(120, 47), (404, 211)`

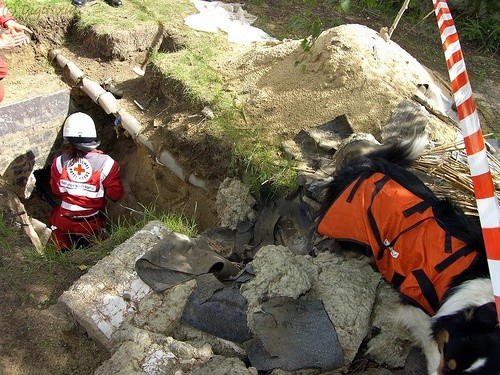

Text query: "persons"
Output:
(71, 0), (123, 8)
(0, 1), (33, 103)
(50, 112), (125, 255)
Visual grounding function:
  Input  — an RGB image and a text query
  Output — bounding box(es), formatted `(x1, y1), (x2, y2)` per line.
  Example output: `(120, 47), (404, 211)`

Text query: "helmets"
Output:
(63, 112), (96, 137)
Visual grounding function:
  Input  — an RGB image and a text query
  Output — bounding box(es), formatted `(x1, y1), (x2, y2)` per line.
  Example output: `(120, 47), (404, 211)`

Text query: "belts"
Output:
(63, 212), (100, 222)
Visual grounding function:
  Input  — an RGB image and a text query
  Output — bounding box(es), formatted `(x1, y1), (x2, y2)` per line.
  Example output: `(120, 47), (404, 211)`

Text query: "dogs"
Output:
(313, 128), (500, 375)
